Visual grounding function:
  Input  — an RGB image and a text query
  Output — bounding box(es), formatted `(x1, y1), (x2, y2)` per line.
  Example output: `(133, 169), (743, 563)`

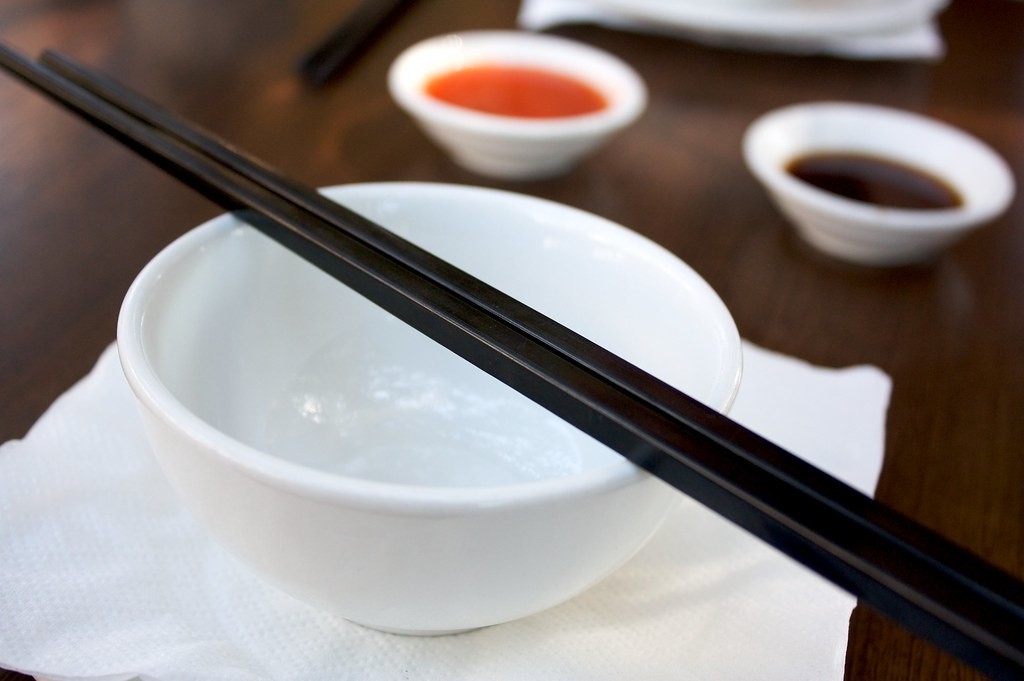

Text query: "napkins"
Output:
(0, 337), (894, 681)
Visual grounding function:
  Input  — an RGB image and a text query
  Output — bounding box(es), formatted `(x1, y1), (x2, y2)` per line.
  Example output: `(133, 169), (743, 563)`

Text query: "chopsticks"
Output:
(292, 0), (416, 85)
(1, 48), (1024, 680)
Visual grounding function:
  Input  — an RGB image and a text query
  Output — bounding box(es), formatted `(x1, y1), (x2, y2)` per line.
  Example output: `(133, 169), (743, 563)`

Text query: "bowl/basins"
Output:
(117, 179), (744, 637)
(386, 28), (649, 178)
(741, 100), (1016, 266)
